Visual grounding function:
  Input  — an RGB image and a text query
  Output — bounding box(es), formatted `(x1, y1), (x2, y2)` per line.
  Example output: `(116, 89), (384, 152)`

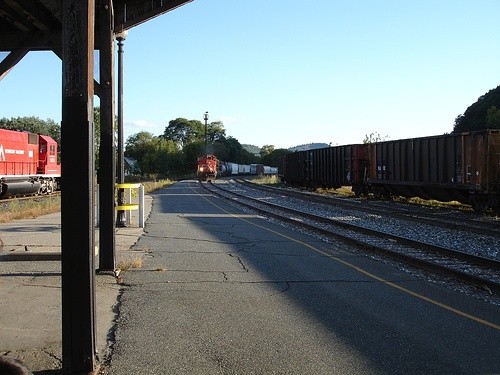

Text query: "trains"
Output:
(278, 130), (499, 214)
(197, 153), (278, 179)
(0, 127), (61, 193)
